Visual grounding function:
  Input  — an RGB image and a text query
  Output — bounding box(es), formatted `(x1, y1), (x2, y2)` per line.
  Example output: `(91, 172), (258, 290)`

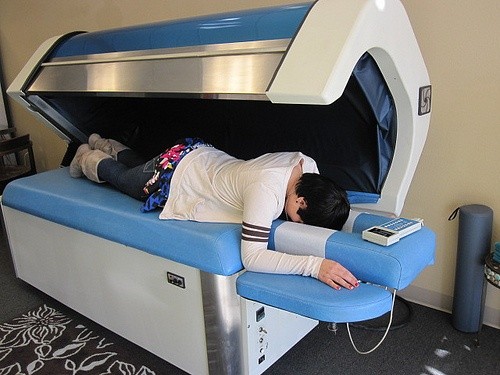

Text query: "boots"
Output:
(70, 144), (114, 183)
(89, 134), (130, 161)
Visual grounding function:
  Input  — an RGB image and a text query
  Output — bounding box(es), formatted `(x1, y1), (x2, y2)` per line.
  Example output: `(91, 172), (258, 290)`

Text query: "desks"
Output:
(473, 252), (500, 348)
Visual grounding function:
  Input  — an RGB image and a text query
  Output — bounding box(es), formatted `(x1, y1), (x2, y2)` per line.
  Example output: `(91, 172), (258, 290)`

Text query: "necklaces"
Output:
(285, 194), (290, 221)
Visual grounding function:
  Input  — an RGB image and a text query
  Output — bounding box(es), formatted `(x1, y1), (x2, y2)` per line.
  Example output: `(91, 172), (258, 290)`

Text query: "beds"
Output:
(0, 164), (435, 375)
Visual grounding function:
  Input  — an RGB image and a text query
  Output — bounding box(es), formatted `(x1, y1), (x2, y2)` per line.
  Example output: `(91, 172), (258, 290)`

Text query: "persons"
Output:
(71, 133), (361, 291)
